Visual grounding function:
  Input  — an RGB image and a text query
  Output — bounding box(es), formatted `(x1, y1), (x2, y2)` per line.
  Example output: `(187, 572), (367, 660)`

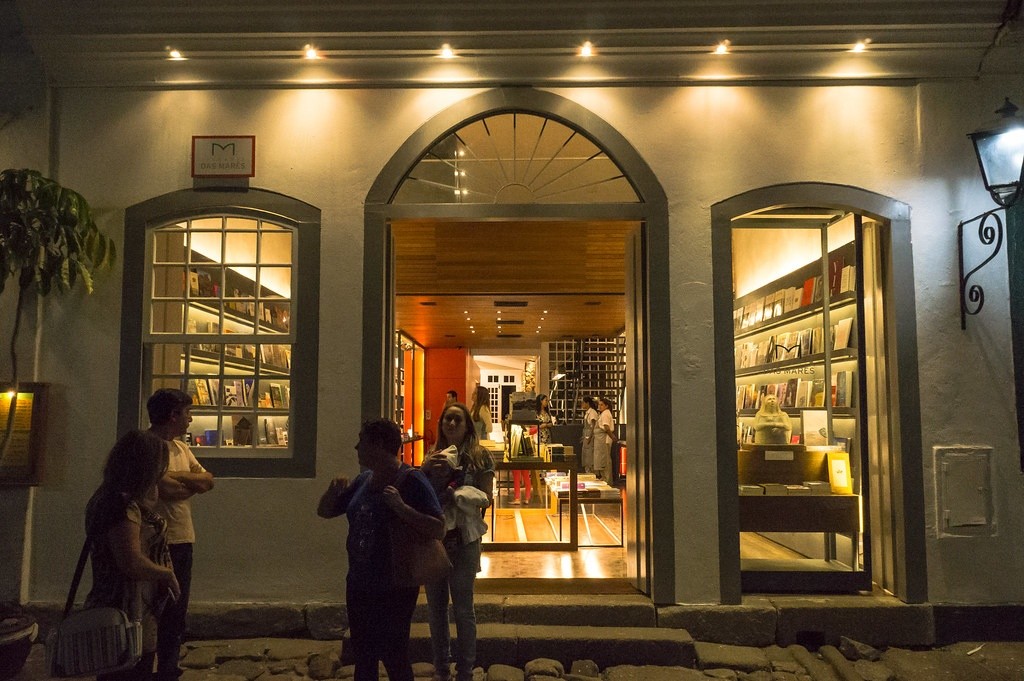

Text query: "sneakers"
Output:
(508, 500), (520, 504)
(523, 499), (528, 504)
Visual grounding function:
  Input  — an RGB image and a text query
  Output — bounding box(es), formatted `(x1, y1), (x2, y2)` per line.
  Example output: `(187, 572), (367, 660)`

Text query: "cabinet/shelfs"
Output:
(395, 345), (404, 430)
(539, 336), (626, 425)
(181, 245), (289, 447)
(740, 494), (859, 571)
(732, 239), (859, 494)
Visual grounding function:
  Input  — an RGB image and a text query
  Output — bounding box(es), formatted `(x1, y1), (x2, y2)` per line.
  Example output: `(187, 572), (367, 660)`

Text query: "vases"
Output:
(0, 601), (37, 678)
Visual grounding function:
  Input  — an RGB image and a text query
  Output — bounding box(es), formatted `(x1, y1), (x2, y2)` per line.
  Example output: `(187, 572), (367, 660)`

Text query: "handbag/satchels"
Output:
(48, 606), (144, 679)
(389, 519), (455, 587)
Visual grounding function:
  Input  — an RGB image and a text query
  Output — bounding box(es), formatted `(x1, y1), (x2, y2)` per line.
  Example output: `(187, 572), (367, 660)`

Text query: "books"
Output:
(479, 440), (504, 464)
(733, 264), (858, 495)
(179, 263), (404, 460)
(504, 390), (536, 459)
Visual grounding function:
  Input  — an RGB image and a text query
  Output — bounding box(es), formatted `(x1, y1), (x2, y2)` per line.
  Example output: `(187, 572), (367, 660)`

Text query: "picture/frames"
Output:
(826, 452), (853, 494)
(1, 382), (50, 486)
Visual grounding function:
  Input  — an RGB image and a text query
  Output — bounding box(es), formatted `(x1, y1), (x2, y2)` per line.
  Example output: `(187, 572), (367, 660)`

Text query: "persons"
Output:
(508, 469), (532, 505)
(316, 418), (443, 681)
(85, 429), (181, 680)
(535, 393), (553, 483)
(142, 388), (215, 681)
(446, 390), (457, 404)
(419, 401), (496, 681)
(469, 386), (493, 440)
(594, 398), (619, 488)
(544, 443), (620, 500)
(581, 396), (602, 479)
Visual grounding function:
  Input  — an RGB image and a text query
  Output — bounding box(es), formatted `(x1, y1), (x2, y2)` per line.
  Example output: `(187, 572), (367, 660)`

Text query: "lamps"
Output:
(967, 97), (1024, 208)
